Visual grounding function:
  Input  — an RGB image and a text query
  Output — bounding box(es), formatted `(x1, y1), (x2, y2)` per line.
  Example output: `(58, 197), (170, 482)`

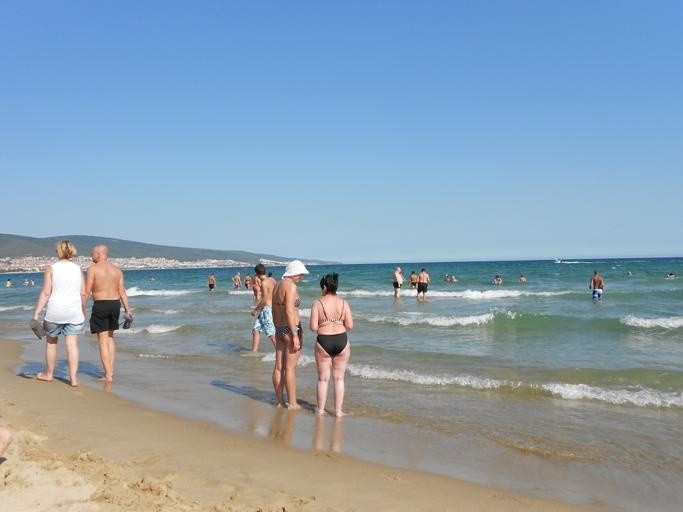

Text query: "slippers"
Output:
(31, 328), (42, 340)
(29, 319), (46, 337)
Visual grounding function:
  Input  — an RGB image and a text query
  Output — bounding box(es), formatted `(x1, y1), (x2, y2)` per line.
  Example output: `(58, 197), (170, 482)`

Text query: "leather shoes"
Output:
(122, 312), (134, 323)
(122, 321), (131, 328)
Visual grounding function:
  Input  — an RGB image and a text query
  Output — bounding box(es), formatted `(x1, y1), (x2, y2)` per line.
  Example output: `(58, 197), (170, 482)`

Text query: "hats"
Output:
(282, 260), (310, 279)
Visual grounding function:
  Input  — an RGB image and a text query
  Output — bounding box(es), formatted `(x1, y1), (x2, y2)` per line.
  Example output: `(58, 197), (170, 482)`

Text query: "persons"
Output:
(267, 272), (275, 285)
(312, 414), (343, 453)
(415, 268), (430, 301)
(238, 263), (277, 356)
(408, 271), (418, 289)
(398, 272), (404, 286)
(85, 243), (130, 384)
(392, 266), (402, 299)
(268, 408), (296, 450)
(207, 272), (216, 291)
(233, 270), (240, 289)
(270, 258), (308, 411)
(244, 272), (251, 289)
(308, 271), (354, 417)
(492, 275), (501, 285)
(30, 238), (87, 388)
(518, 273), (527, 282)
(444, 274), (458, 282)
(588, 270), (605, 304)
